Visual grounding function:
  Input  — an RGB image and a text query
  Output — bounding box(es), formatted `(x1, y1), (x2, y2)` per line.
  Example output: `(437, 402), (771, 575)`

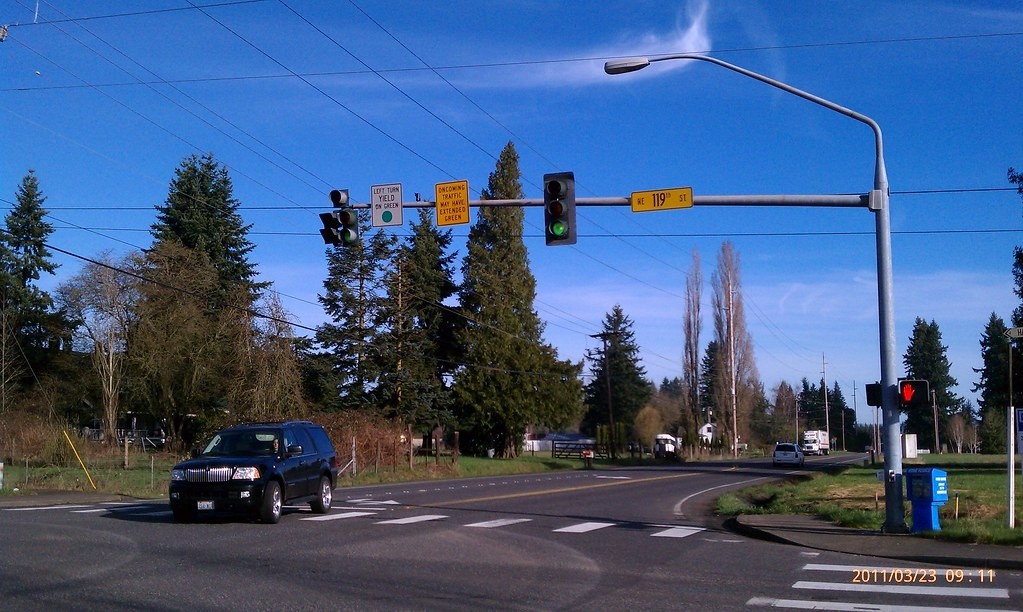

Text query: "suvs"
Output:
(169, 420), (337, 524)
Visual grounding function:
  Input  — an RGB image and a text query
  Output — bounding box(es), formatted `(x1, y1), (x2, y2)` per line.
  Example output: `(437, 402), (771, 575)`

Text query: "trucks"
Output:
(802, 430), (830, 456)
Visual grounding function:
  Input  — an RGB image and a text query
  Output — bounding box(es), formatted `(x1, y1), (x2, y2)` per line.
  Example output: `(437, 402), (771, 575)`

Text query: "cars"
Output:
(84, 428), (168, 453)
(773, 442), (805, 467)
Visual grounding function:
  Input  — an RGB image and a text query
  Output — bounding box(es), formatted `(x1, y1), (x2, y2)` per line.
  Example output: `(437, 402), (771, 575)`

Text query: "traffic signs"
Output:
(370, 181), (404, 228)
(434, 179), (470, 227)
(1003, 327), (1023, 337)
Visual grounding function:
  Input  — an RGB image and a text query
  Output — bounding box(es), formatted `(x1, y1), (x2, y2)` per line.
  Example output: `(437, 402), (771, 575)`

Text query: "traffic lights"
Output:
(338, 210), (359, 247)
(898, 380), (931, 403)
(330, 189), (350, 207)
(318, 211), (340, 247)
(544, 172), (579, 246)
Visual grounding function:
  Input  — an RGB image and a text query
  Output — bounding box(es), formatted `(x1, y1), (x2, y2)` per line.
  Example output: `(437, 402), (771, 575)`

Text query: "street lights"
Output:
(604, 54), (907, 535)
(707, 407), (712, 424)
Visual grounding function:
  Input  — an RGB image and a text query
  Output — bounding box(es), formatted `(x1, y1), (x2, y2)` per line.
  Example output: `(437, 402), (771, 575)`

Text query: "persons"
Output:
(269, 438), (278, 453)
